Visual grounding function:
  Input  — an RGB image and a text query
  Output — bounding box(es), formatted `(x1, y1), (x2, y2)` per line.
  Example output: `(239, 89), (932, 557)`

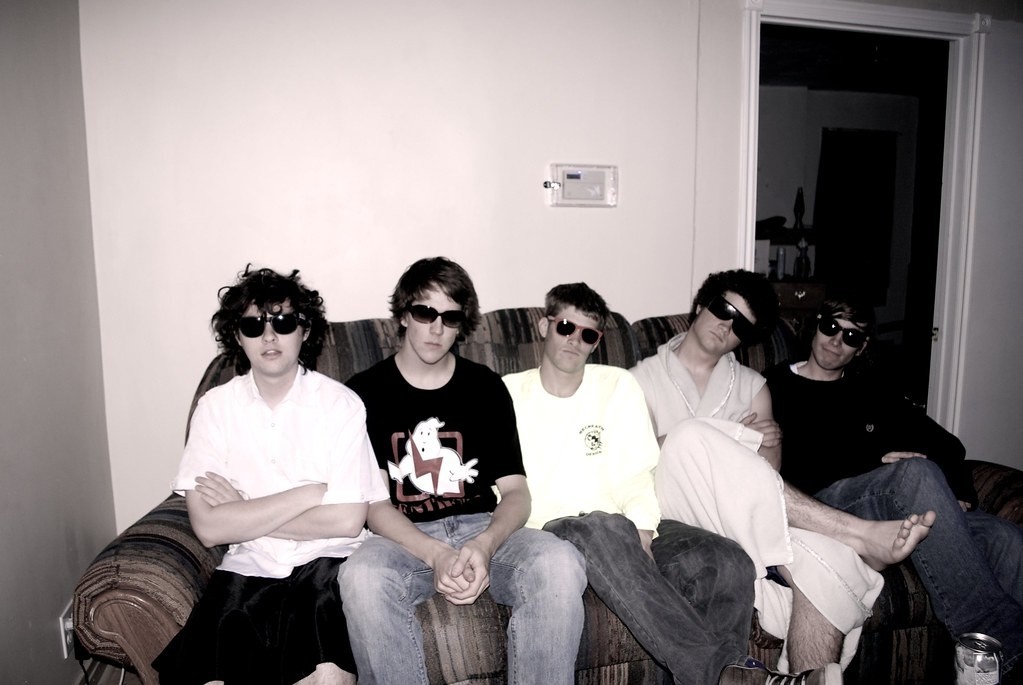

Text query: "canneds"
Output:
(953, 632), (1004, 685)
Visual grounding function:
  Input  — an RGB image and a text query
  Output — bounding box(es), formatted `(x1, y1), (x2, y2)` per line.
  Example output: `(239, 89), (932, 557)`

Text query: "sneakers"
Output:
(718, 662), (843, 685)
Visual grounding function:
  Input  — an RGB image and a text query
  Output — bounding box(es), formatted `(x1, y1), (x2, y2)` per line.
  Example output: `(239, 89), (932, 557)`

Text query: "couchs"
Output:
(73, 308), (1023, 685)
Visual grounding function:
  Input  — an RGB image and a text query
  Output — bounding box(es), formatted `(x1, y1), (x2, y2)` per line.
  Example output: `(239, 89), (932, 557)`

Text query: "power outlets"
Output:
(58, 600), (74, 659)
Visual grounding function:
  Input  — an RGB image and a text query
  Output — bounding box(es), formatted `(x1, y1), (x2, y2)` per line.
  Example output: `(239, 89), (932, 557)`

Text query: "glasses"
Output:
(549, 315), (603, 346)
(818, 312), (867, 348)
(232, 311), (311, 338)
(404, 303), (469, 328)
(706, 294), (758, 343)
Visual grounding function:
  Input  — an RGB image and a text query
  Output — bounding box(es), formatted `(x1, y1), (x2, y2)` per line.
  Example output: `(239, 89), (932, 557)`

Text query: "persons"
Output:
(628, 269), (936, 675)
(500, 282), (844, 685)
(760, 287), (1023, 685)
(151, 263), (391, 685)
(337, 256), (587, 685)
